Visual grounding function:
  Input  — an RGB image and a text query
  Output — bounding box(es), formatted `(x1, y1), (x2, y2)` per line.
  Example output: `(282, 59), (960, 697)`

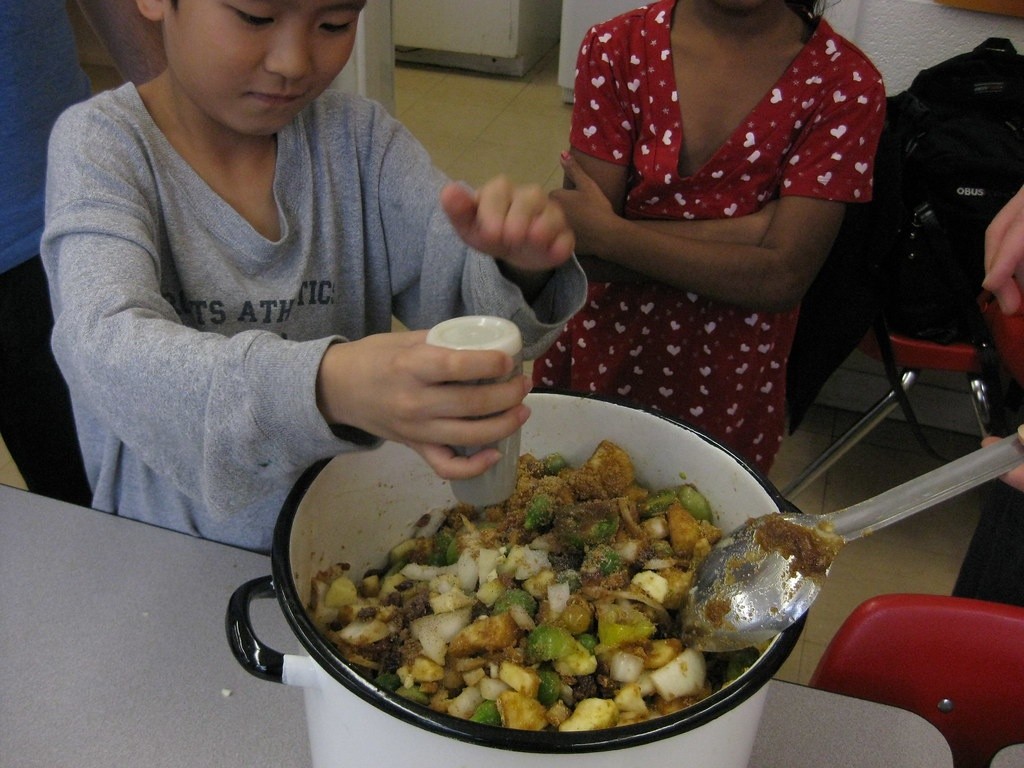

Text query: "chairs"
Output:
(807, 593), (1024, 768)
(783, 326), (998, 506)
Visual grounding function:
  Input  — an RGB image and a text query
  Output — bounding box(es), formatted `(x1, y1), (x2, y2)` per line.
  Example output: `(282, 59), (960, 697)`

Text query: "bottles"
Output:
(421, 313), (527, 510)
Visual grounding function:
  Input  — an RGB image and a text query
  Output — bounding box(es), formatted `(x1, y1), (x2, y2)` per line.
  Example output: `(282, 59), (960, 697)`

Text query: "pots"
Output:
(223, 389), (825, 768)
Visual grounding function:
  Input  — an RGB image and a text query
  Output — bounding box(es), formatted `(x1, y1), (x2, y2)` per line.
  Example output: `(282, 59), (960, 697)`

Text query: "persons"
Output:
(0, 1), (167, 509)
(532, 0), (885, 478)
(40, 0), (589, 557)
(950, 185), (1024, 768)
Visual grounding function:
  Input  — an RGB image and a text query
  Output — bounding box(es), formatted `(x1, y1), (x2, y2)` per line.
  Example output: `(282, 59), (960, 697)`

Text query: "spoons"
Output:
(682, 430), (1024, 652)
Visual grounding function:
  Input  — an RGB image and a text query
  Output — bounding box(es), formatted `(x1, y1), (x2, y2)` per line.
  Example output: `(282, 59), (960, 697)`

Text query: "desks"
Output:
(0, 484), (954, 768)
